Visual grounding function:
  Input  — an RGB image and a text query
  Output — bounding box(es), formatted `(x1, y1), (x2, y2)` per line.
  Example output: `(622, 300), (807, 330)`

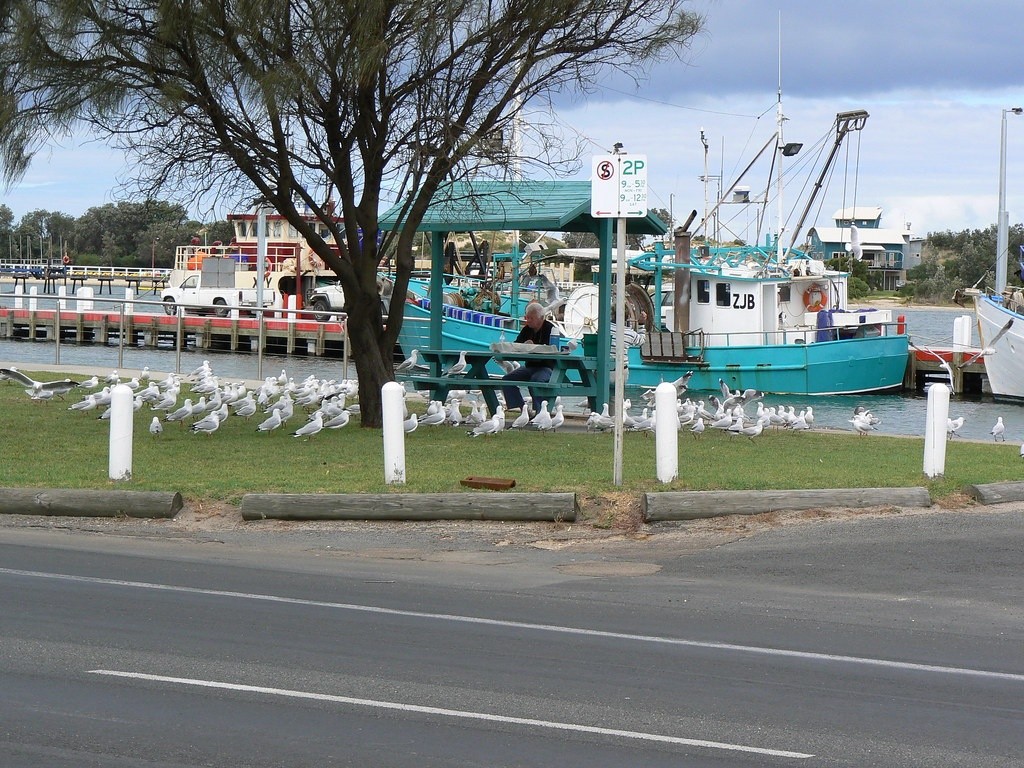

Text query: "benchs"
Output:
(407, 372), (612, 419)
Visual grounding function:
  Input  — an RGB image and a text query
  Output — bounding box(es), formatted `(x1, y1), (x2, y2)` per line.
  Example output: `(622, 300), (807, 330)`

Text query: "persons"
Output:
(502, 303), (569, 420)
(594, 305), (647, 384)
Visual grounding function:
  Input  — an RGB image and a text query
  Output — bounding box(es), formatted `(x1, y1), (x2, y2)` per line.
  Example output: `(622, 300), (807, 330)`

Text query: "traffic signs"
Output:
(617, 154), (647, 218)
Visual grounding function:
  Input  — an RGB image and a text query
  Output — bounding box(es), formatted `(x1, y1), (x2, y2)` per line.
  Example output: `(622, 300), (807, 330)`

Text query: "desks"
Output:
(414, 347), (616, 412)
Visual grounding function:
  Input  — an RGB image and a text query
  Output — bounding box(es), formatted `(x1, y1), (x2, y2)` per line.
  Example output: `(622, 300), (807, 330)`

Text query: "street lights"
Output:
(994, 106), (1024, 297)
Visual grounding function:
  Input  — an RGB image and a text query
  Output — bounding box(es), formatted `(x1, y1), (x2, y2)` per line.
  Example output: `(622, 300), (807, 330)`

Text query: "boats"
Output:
(166, 6), (909, 398)
(953, 226), (1024, 407)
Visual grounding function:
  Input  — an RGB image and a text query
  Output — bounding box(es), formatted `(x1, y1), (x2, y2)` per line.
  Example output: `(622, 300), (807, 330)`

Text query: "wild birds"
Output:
(947, 416), (968, 442)
(537, 273), (575, 321)
(845, 225), (864, 260)
(0, 345), (816, 449)
(990, 416), (1007, 443)
(848, 405), (883, 439)
(1019, 439), (1024, 464)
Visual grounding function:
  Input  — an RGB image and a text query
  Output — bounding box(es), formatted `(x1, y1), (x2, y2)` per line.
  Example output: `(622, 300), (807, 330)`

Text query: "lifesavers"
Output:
(803, 282), (827, 312)
(63, 256), (69, 263)
(265, 257), (273, 272)
(475, 292), (500, 314)
(309, 249), (324, 267)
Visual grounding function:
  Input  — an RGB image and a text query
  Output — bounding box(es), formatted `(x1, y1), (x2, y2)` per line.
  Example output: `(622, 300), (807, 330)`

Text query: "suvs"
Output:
(308, 278), (388, 324)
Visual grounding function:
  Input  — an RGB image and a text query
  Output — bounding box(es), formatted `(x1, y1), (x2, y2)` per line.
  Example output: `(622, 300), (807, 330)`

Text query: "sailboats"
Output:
(0, 233), (68, 279)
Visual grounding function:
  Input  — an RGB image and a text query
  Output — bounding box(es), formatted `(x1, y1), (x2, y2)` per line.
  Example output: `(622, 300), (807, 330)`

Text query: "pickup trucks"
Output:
(160, 274), (276, 317)
